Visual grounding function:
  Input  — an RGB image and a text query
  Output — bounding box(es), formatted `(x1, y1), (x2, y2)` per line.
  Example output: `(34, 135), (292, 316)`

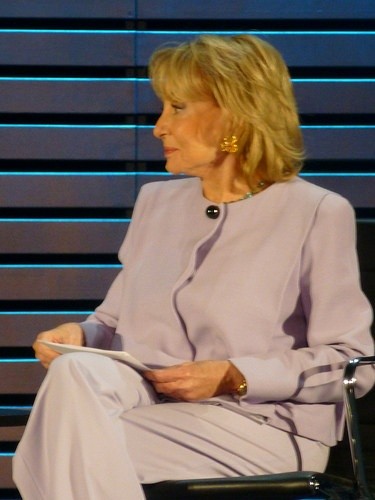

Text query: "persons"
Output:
(11, 35), (375, 500)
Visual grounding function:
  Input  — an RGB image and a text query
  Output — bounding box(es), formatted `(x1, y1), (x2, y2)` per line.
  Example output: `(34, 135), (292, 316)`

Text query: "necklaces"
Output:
(225, 180), (266, 203)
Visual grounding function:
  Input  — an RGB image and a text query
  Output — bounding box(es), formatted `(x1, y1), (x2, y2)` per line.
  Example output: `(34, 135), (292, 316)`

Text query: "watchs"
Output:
(234, 380), (247, 396)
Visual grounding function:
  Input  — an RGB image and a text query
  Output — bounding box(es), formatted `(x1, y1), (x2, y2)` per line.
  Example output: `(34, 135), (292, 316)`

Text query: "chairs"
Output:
(142, 221), (375, 500)
